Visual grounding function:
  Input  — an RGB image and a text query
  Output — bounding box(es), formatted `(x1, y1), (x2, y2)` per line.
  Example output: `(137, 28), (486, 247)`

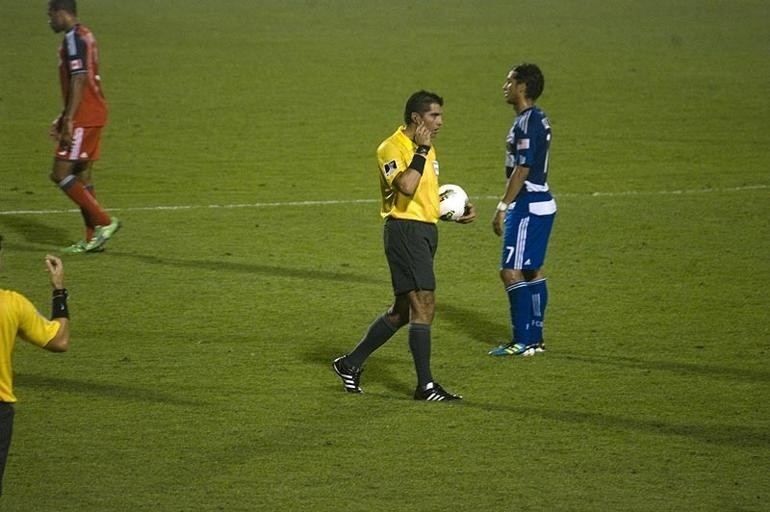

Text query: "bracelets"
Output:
(414, 144), (431, 155)
(495, 200), (509, 213)
(48, 297), (71, 321)
(407, 153), (427, 178)
(51, 287), (70, 297)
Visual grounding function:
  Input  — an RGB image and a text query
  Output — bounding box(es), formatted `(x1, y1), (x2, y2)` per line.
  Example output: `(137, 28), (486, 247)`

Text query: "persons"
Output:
(47, 0), (123, 256)
(0, 233), (73, 512)
(331, 87), (478, 403)
(485, 60), (559, 360)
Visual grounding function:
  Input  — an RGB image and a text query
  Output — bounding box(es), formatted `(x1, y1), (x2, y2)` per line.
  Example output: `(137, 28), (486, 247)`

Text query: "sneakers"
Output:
(66, 241), (105, 255)
(87, 216), (120, 253)
(488, 343), (547, 359)
(413, 382), (461, 403)
(330, 354), (364, 393)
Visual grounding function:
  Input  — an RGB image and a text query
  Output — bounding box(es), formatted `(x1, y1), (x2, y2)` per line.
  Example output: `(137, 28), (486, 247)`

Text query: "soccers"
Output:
(436, 184), (469, 223)
